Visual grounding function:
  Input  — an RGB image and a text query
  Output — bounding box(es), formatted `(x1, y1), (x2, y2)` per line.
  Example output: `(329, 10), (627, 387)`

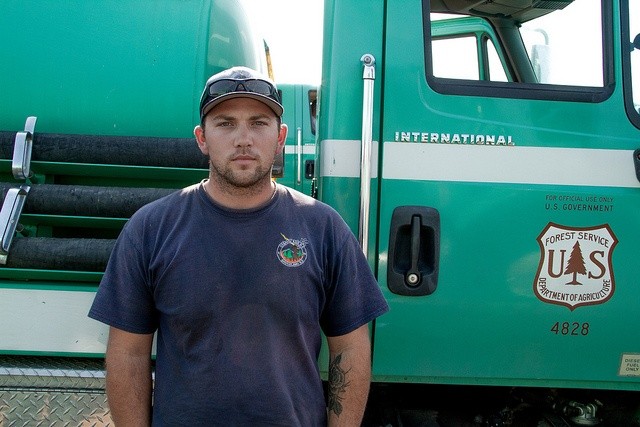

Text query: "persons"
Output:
(86, 65), (389, 426)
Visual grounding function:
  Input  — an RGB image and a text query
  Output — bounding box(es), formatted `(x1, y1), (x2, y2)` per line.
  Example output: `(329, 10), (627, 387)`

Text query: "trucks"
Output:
(0, 0), (640, 426)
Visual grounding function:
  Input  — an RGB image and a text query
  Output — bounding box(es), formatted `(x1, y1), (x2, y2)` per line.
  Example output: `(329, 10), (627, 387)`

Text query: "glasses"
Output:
(201, 80), (279, 109)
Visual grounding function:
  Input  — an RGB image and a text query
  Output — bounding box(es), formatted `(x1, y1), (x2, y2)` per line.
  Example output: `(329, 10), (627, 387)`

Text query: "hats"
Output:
(199, 67), (283, 116)
